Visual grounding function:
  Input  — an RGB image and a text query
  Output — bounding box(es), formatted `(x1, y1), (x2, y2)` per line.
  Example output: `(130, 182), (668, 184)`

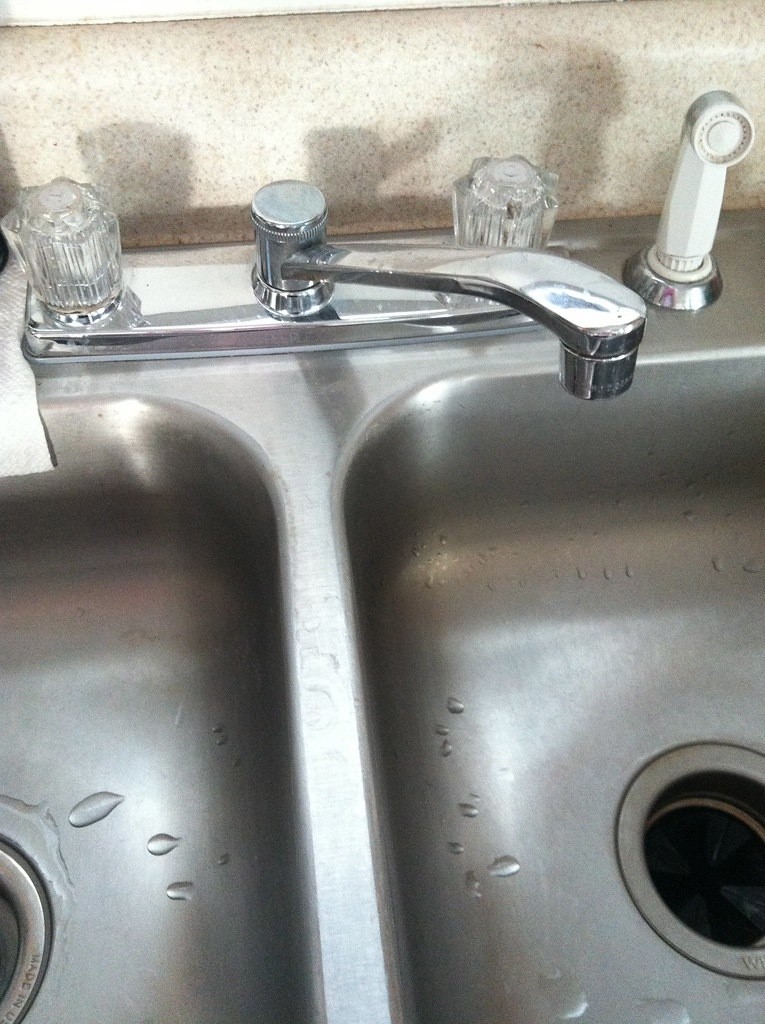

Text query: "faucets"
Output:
(626, 87), (756, 316)
(245, 178), (649, 400)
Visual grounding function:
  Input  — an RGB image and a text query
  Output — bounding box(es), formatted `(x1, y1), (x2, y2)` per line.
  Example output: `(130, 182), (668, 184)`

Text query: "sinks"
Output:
(271, 339), (765, 1024)
(0, 376), (369, 1024)
